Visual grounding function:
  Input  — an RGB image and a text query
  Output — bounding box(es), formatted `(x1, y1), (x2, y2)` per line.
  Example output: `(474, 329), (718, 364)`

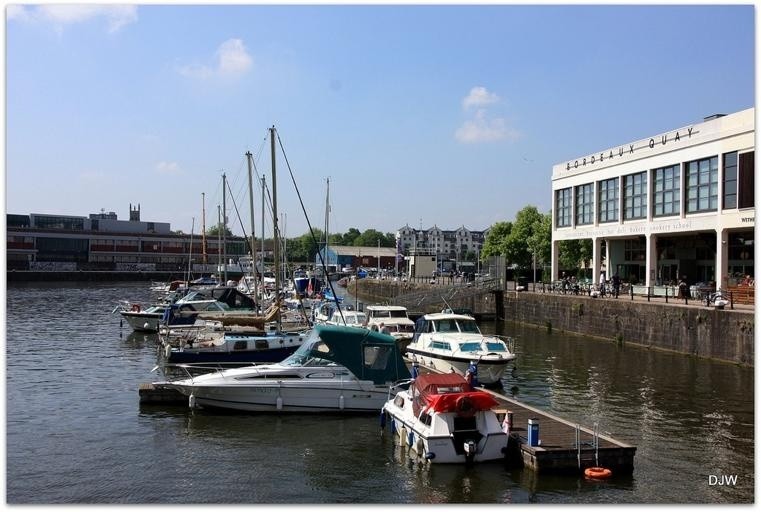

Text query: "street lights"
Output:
(473, 247), (479, 281)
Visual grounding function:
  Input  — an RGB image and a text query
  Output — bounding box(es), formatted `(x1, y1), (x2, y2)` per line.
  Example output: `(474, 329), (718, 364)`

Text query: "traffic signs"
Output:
(374, 367), (515, 468)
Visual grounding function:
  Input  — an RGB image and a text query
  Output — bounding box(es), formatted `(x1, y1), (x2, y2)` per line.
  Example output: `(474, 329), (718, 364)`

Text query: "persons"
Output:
(547, 270), (626, 299)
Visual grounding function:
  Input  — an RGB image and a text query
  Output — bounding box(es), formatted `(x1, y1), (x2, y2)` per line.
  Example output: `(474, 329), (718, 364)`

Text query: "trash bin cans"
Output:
(336, 272), (343, 281)
(518, 277), (528, 292)
(358, 303), (363, 312)
(527, 418), (540, 446)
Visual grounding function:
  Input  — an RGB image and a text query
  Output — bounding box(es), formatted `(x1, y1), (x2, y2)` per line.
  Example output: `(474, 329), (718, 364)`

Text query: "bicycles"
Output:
(700, 287), (729, 307)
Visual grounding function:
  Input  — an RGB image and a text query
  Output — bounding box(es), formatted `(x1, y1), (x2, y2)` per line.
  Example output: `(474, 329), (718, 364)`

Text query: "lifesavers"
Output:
(132, 304), (140, 312)
(456, 396), (478, 418)
(585, 467), (613, 478)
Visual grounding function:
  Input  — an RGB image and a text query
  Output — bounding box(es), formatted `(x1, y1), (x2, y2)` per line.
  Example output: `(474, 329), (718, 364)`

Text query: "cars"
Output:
(453, 270), (488, 278)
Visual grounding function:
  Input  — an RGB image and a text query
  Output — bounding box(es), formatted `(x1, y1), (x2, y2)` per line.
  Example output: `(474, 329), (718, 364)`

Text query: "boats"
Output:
(399, 293), (515, 387)
(150, 323), (414, 421)
